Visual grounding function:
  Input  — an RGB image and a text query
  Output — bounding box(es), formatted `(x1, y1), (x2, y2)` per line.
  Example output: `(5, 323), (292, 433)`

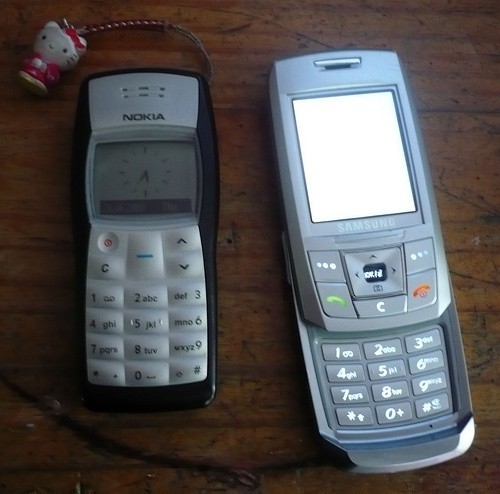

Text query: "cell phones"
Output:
(70, 67), (219, 413)
(267, 50), (476, 475)
